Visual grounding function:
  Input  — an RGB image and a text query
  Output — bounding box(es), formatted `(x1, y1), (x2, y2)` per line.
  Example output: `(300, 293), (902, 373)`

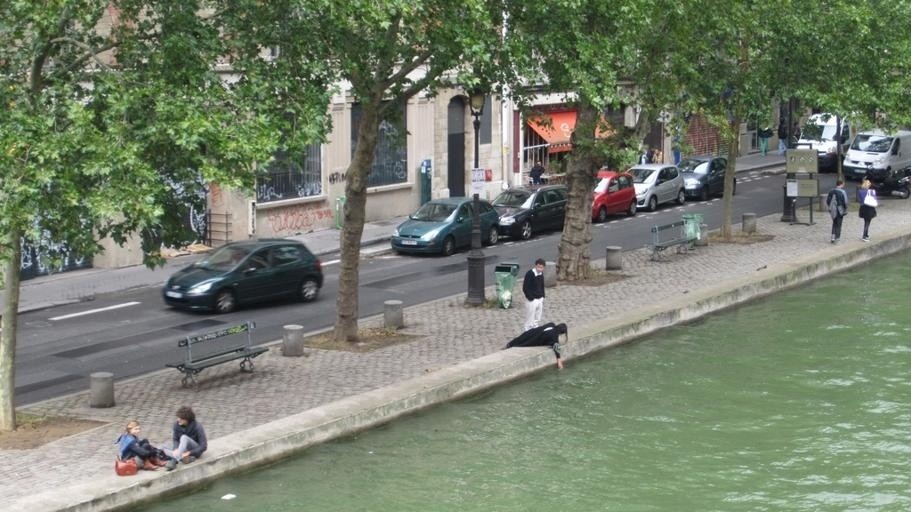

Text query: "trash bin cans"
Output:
(681, 213), (705, 250)
(494, 262), (519, 309)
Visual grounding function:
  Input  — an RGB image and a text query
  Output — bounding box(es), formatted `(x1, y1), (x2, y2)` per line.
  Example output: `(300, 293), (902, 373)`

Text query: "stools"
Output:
(543, 261), (558, 288)
(282, 324), (306, 356)
(383, 299), (405, 330)
(89, 371), (117, 409)
(607, 245), (623, 271)
(694, 223), (710, 246)
(819, 193), (830, 211)
(742, 212), (758, 234)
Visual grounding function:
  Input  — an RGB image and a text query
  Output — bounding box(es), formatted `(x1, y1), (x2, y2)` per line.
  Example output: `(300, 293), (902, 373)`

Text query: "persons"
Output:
(530, 161), (545, 184)
(826, 180), (849, 242)
(523, 258), (546, 332)
(857, 179), (878, 242)
(507, 323), (568, 369)
(640, 147), (681, 168)
(758, 119), (773, 157)
(164, 405), (208, 471)
(793, 120), (802, 141)
(114, 421), (167, 471)
(777, 115), (787, 157)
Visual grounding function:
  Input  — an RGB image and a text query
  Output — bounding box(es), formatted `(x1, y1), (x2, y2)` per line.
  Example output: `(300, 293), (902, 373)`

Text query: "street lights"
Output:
(463, 85), (492, 308)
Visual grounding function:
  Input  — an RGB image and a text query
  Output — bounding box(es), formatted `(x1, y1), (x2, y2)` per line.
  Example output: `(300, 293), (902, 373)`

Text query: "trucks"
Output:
(796, 111), (892, 173)
(839, 128), (911, 182)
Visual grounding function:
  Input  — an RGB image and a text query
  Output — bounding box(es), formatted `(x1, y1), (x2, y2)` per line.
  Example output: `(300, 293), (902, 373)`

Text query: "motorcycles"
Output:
(858, 160), (910, 197)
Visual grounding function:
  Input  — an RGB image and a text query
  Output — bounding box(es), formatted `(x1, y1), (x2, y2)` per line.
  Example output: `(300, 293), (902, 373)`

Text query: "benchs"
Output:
(165, 320), (268, 393)
(642, 219), (698, 262)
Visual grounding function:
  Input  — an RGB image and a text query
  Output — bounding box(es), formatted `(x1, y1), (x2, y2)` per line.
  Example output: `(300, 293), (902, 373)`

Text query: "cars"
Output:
(161, 237), (324, 314)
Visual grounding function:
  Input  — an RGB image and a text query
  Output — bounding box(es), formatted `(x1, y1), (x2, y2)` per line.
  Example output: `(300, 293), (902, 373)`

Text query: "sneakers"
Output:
(182, 455), (196, 464)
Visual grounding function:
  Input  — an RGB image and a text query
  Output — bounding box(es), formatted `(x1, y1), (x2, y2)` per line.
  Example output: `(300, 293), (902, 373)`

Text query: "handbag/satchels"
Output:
(115, 457), (138, 477)
(863, 189), (878, 208)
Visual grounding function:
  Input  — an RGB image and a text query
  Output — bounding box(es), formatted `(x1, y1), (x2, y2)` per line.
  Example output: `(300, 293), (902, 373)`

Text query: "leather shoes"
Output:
(861, 235), (870, 243)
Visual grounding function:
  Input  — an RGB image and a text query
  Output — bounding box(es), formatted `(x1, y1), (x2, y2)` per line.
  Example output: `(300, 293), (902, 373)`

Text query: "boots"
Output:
(143, 457), (159, 470)
(150, 455), (168, 467)
